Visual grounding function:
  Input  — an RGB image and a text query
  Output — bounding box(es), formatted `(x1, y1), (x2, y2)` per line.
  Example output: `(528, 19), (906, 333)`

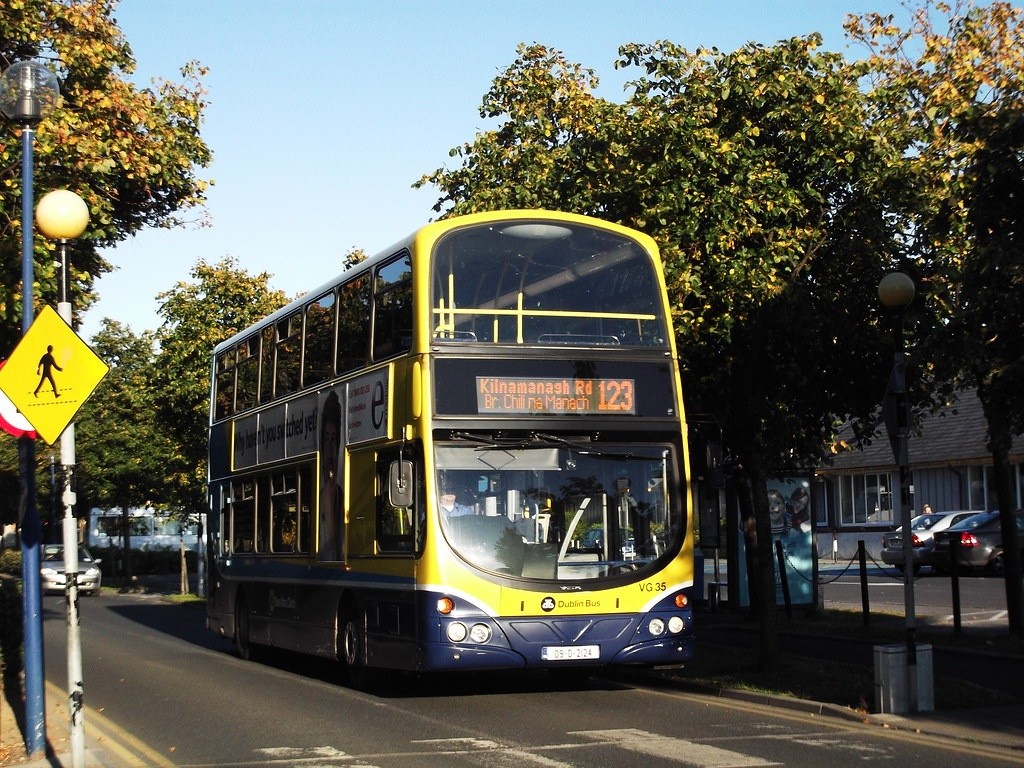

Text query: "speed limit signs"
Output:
(0, 360), (42, 441)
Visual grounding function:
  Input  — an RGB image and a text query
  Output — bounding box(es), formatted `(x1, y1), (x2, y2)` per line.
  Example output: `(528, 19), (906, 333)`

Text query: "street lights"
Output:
(871, 269), (926, 718)
(29, 187), (91, 767)
(0, 59), (62, 758)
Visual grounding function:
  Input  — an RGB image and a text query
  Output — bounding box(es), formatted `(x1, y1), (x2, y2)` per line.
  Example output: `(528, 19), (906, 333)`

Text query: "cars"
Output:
(38, 543), (103, 597)
(932, 509), (1022, 576)
(880, 508), (986, 573)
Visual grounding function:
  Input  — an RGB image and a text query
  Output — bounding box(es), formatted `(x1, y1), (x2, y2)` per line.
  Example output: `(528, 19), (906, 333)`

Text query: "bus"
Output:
(195, 208), (707, 685)
(87, 505), (206, 577)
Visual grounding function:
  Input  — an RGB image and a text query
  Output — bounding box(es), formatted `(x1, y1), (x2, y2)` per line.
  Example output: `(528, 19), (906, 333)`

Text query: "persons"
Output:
(785, 487), (809, 525)
(923, 505), (932, 514)
(767, 489), (790, 537)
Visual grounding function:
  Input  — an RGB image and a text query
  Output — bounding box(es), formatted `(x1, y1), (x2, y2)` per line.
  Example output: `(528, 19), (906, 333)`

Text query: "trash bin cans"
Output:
(872, 641), (936, 718)
